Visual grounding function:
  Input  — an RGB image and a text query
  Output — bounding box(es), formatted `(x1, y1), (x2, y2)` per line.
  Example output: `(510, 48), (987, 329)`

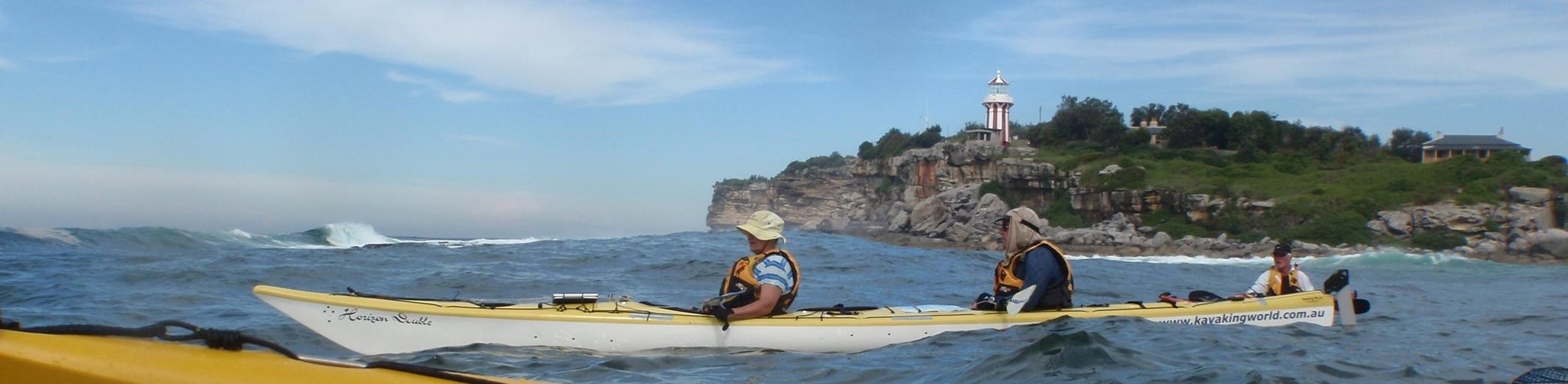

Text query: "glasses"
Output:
(1002, 223), (1009, 232)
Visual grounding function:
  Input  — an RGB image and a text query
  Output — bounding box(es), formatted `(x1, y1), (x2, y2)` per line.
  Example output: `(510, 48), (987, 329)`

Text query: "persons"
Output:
(698, 210), (802, 324)
(1244, 242), (1316, 298)
(970, 206), (1075, 316)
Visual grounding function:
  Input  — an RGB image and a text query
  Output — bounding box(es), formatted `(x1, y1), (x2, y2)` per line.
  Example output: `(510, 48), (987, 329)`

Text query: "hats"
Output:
(736, 210), (788, 243)
(1271, 244), (1291, 256)
(994, 215), (1009, 226)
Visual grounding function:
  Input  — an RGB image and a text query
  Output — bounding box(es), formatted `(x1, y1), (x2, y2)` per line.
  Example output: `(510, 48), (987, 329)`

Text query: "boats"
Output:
(251, 266), (1373, 355)
(1, 321), (563, 384)
